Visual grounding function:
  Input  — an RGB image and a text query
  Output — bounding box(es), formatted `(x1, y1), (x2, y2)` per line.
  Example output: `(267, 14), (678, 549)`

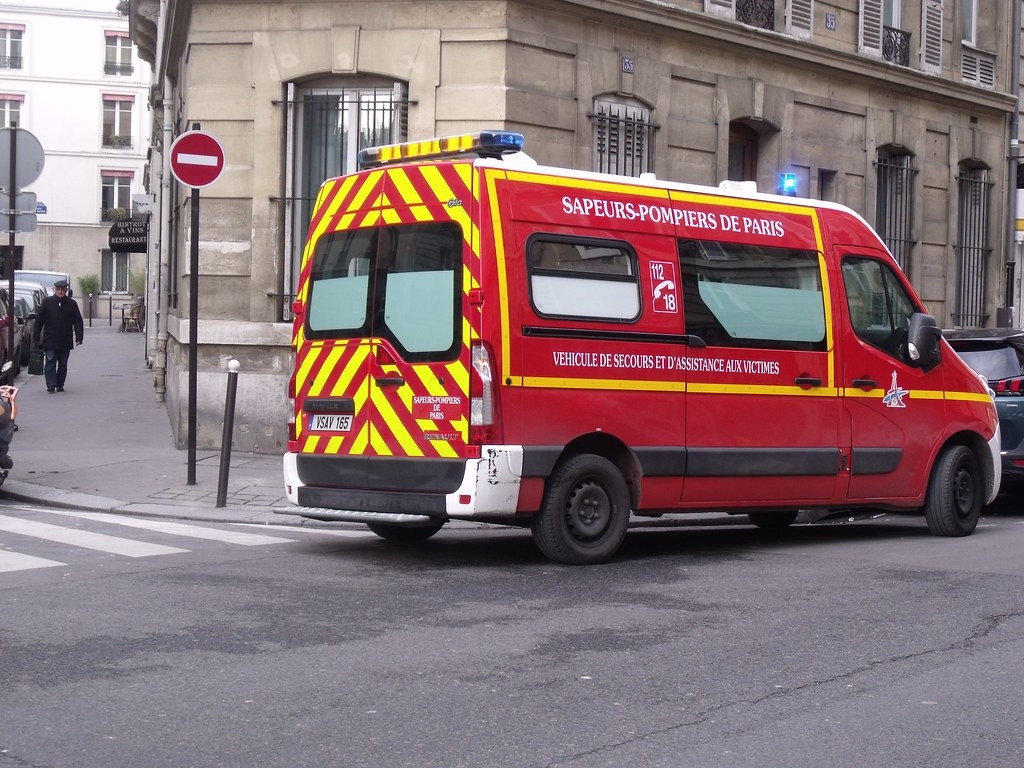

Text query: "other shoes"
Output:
(47, 384), (55, 391)
(57, 386), (64, 392)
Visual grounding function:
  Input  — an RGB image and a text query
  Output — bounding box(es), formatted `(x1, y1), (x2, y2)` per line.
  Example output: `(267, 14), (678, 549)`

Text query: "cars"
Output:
(938, 328), (1024, 517)
(13, 295), (37, 345)
(0, 279), (49, 297)
(14, 269), (73, 299)
(6, 289), (40, 312)
(0, 284), (45, 307)
(0, 287), (24, 383)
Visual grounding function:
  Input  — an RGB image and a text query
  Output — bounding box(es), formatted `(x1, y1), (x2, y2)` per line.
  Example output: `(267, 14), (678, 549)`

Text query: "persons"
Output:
(33, 280), (84, 392)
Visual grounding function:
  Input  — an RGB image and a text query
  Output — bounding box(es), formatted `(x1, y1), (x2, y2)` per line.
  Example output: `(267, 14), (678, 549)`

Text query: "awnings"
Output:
(108, 219), (147, 253)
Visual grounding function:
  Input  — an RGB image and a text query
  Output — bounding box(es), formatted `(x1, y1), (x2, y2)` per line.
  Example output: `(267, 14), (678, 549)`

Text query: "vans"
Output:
(282, 130), (1002, 565)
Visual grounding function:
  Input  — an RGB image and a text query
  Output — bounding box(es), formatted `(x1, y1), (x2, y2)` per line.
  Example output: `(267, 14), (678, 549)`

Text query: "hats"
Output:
(54, 280), (69, 287)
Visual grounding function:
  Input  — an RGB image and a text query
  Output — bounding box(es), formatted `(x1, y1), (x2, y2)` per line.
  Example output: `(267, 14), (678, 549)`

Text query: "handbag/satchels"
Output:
(28, 341), (45, 375)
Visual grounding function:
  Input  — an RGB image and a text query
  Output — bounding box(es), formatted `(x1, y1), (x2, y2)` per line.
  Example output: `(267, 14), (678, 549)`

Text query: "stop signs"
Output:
(167, 130), (225, 188)
